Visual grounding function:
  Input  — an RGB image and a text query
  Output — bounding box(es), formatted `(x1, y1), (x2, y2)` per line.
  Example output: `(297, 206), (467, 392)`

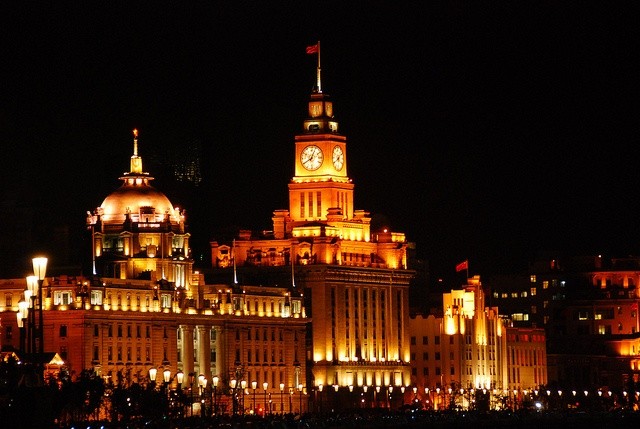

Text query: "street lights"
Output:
(376, 386), (382, 429)
(546, 390), (550, 429)
(26, 273), (38, 384)
(424, 388), (432, 428)
(33, 253), (49, 427)
(363, 385), (368, 429)
(412, 387), (419, 428)
(318, 384), (324, 429)
(23, 290), (33, 429)
(15, 310), (27, 426)
(162, 369), (171, 427)
(350, 385), (354, 429)
(198, 374), (206, 429)
(513, 390), (518, 429)
(470, 388), (475, 429)
(389, 386), (395, 428)
(598, 391), (602, 429)
(493, 388), (498, 429)
(534, 390), (539, 429)
(608, 391), (612, 429)
(436, 388), (442, 428)
(241, 380), (247, 429)
(230, 379), (237, 427)
(263, 381), (268, 428)
(176, 371), (185, 429)
(213, 376), (219, 428)
(483, 389), (489, 428)
(17, 299), (30, 429)
(447, 388), (454, 429)
(583, 391), (590, 429)
(252, 380), (256, 428)
(401, 387), (407, 429)
(335, 384), (341, 429)
(279, 383), (285, 429)
(460, 388), (466, 429)
(503, 388), (508, 429)
(635, 391), (640, 429)
(572, 390), (577, 429)
(623, 391), (628, 429)
(148, 367), (157, 426)
(523, 390), (527, 429)
(558, 390), (563, 428)
(297, 383), (305, 428)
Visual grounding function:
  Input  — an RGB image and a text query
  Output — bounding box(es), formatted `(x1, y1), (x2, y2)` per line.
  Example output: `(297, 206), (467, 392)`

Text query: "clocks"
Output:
(300, 146), (324, 172)
(332, 143), (344, 172)
